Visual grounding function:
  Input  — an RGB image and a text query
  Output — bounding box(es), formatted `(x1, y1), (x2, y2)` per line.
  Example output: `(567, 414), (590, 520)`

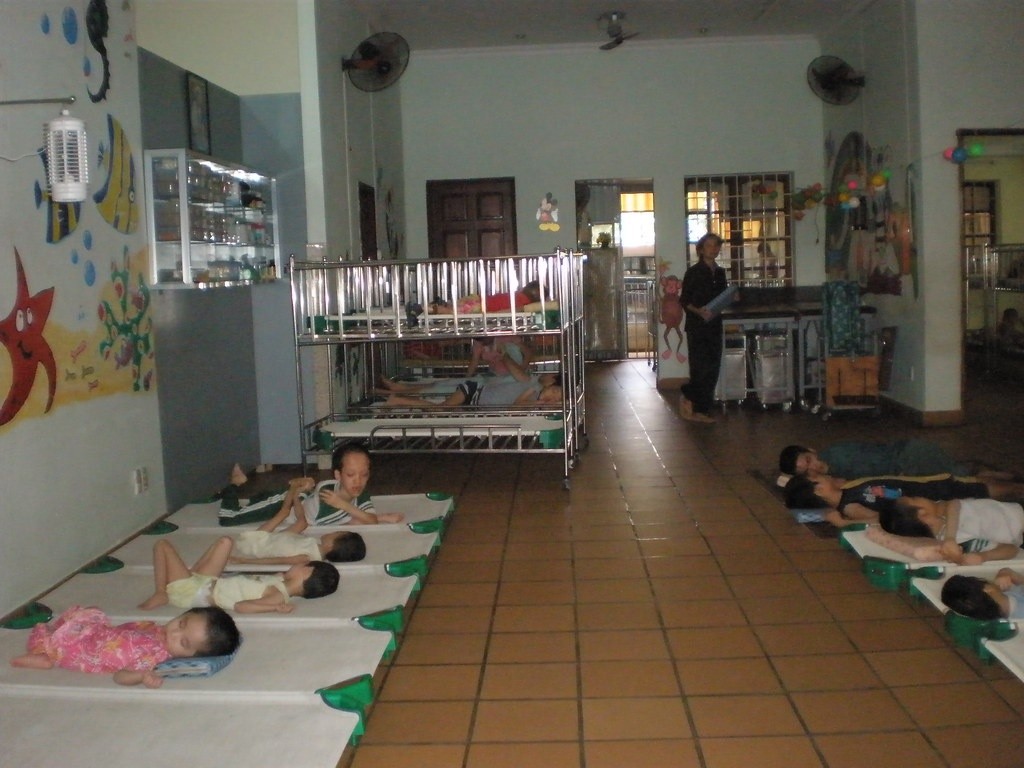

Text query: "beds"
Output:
(757, 461), (1024, 713)
(0, 247), (589, 768)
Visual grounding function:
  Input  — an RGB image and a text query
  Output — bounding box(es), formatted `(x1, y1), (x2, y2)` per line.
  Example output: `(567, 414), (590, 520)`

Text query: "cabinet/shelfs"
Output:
(145, 148), (282, 288)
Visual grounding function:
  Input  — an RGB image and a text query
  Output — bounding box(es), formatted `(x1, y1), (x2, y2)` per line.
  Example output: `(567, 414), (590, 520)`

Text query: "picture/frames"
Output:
(185, 70), (212, 155)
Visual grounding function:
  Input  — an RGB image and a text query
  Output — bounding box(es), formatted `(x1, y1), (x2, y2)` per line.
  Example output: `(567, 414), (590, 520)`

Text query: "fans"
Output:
(341, 31), (411, 92)
(598, 11), (642, 51)
(807, 54), (866, 105)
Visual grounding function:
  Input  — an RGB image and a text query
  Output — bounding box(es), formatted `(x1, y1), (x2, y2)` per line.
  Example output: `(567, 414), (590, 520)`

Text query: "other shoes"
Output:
(692, 413), (716, 424)
(678, 394), (692, 420)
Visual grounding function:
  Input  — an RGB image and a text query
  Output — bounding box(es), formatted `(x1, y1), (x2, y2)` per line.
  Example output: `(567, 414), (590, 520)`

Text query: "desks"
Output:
(717, 301), (823, 408)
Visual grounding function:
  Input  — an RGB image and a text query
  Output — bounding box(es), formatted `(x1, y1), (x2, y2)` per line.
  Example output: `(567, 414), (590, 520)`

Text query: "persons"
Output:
(141, 536), (339, 614)
(998, 308), (1018, 336)
(422, 280), (546, 314)
(375, 336), (568, 411)
(679, 233), (739, 423)
(757, 242), (778, 278)
(230, 477), (366, 564)
(780, 440), (1024, 628)
(218, 441), (403, 527)
(11, 606), (241, 688)
